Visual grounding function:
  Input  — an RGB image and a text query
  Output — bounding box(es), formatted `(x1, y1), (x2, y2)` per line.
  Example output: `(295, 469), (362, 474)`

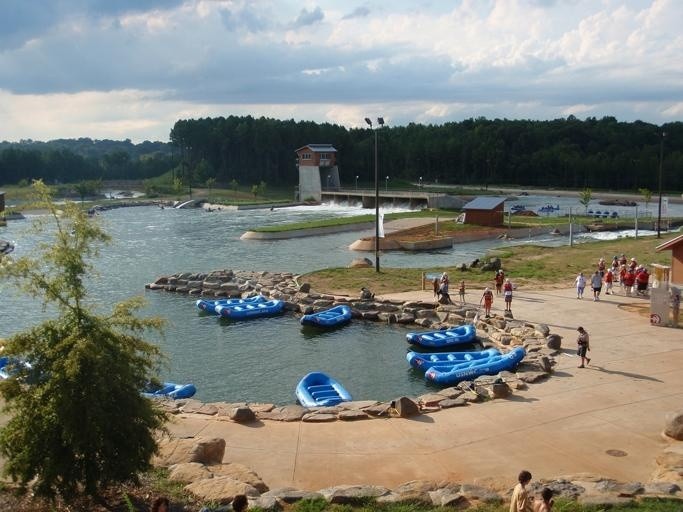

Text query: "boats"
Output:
(70, 222), (82, 235)
(0, 356), (42, 382)
(86, 208), (95, 218)
(404, 323), (475, 348)
(299, 303), (353, 330)
(404, 348), (500, 372)
(217, 297), (286, 320)
(423, 346), (526, 384)
(510, 192), (616, 217)
(196, 294), (267, 314)
(138, 378), (197, 401)
(295, 370), (352, 408)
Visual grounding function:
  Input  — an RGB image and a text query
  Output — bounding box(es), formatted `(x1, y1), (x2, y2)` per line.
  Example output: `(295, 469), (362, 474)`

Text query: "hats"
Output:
(579, 253), (648, 275)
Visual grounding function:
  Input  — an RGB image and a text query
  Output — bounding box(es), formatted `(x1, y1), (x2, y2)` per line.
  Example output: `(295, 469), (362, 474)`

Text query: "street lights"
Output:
(631, 159), (641, 195)
(174, 136), (185, 182)
(650, 128), (667, 237)
(355, 175), (359, 192)
(384, 176), (390, 194)
(186, 144), (193, 198)
(326, 174), (331, 192)
(419, 176), (422, 192)
(363, 116), (386, 274)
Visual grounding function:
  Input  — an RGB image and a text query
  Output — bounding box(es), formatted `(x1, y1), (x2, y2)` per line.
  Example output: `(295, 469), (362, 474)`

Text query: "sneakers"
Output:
(578, 358), (590, 368)
(485, 291), (511, 318)
(577, 291), (648, 302)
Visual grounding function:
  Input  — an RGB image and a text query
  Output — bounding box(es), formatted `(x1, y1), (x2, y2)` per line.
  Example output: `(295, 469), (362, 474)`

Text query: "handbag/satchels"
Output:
(577, 337), (582, 344)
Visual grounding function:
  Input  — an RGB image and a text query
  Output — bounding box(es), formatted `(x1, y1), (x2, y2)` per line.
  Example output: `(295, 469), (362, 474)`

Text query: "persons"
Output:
(510, 470), (533, 511)
(503, 278), (516, 311)
(531, 488), (554, 512)
(198, 507), (211, 512)
(150, 496), (169, 512)
(572, 254), (650, 301)
(431, 272), (456, 305)
(494, 272), (502, 295)
(458, 281), (465, 302)
(233, 495), (248, 511)
(577, 326), (591, 368)
(499, 270), (506, 286)
(480, 287), (493, 318)
